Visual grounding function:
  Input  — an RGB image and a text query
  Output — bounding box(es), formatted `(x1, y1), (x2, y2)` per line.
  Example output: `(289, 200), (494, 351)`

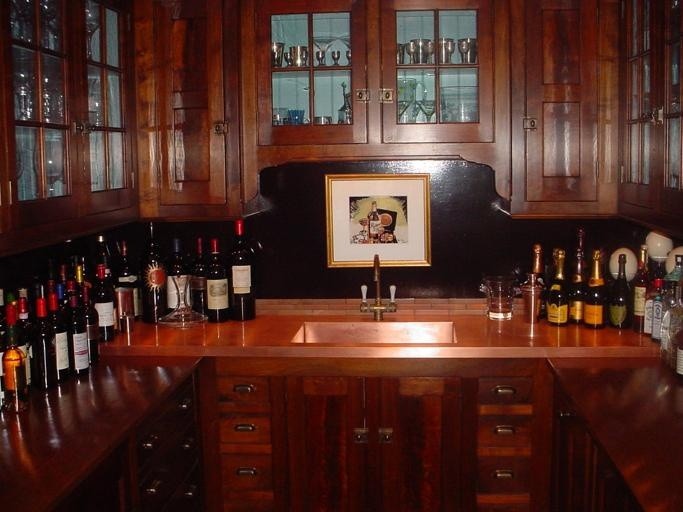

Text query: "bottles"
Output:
(526, 225), (683, 382)
(0, 221), (256, 415)
(366, 200), (381, 241)
(521, 273), (542, 326)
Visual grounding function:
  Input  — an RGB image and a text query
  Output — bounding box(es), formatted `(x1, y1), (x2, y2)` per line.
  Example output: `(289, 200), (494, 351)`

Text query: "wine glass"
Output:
(395, 34), (478, 125)
(7, 0), (111, 199)
(358, 217), (368, 235)
(269, 29), (354, 126)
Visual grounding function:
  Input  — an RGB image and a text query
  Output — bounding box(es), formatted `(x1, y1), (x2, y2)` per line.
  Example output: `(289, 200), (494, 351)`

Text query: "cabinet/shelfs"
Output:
(550, 381), (638, 509)
(121, 371), (207, 512)
(509, 1), (620, 219)
(464, 373), (548, 512)
(137, 0), (243, 229)
(253, 1), (496, 150)
(621, 1), (682, 237)
(200, 373), (284, 511)
(0, 1), (137, 255)
(284, 374), (462, 512)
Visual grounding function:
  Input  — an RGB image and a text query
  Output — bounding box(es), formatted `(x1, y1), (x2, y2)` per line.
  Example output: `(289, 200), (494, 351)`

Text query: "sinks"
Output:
(291, 321), (457, 344)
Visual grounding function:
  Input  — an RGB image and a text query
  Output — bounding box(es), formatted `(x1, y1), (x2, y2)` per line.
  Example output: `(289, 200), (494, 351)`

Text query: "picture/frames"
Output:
(322, 172), (433, 272)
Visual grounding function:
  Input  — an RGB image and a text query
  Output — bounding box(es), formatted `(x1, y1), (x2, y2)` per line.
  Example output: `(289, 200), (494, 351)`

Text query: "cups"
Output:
(478, 273), (518, 321)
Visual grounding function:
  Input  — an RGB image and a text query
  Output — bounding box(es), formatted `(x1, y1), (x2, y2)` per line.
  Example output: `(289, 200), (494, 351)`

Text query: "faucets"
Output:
(373, 254), (382, 304)
